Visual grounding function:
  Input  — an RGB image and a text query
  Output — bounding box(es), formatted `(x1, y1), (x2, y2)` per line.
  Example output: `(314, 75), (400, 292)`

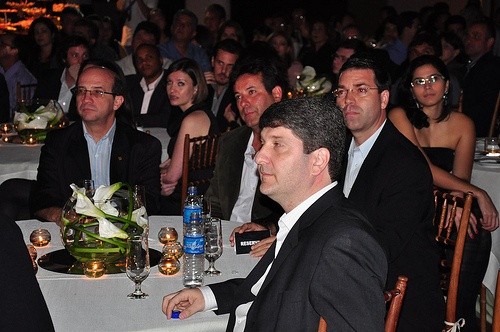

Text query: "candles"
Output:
(29, 227), (51, 272)
(84, 258), (104, 277)
(484, 133), (500, 156)
(157, 226), (182, 275)
(2, 123), (40, 146)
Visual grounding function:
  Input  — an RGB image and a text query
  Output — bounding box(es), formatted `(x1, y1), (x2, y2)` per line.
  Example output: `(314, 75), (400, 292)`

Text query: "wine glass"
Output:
(204, 217), (223, 275)
(125, 235), (151, 299)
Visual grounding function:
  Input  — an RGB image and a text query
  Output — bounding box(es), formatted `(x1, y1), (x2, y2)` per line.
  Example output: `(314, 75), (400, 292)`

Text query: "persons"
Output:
(0, 0), (228, 126)
(202, 0), (418, 127)
(202, 52), (289, 225)
(408, 0), (500, 137)
(333, 52), (449, 332)
(160, 57), (219, 217)
(389, 55), (500, 332)
(162, 97), (388, 332)
(34, 58), (163, 228)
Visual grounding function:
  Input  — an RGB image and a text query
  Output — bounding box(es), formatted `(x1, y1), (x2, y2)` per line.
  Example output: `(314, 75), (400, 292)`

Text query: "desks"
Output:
(0, 126), (172, 189)
(13, 219), (275, 332)
(460, 136), (500, 297)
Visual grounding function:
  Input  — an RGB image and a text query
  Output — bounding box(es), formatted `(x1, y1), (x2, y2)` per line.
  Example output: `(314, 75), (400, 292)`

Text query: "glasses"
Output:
(413, 73), (445, 86)
(76, 86), (114, 99)
(331, 86), (378, 98)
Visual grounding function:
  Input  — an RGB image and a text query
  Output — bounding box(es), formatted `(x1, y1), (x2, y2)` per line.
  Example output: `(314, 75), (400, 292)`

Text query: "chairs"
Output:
(15, 82), (39, 108)
(180, 129), (231, 215)
(318, 91), (500, 331)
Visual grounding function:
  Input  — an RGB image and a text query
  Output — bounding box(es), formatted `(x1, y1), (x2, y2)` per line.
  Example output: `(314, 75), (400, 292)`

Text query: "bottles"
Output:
(182, 213), (204, 288)
(183, 185), (200, 232)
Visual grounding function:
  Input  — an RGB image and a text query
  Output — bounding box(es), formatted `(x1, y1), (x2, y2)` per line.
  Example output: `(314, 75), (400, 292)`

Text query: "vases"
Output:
(61, 183), (148, 266)
(14, 103), (65, 143)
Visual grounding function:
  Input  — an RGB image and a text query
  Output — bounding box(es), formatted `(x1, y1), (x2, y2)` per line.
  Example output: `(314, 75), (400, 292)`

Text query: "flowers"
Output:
(12, 101), (63, 133)
(72, 181), (149, 246)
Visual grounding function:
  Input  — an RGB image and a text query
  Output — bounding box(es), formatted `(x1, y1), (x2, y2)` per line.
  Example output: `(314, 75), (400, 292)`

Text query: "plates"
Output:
(473, 158), (500, 165)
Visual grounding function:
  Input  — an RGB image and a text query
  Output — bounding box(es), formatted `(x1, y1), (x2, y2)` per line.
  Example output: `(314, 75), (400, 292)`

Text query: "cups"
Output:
(198, 196), (211, 222)
(485, 137), (500, 158)
